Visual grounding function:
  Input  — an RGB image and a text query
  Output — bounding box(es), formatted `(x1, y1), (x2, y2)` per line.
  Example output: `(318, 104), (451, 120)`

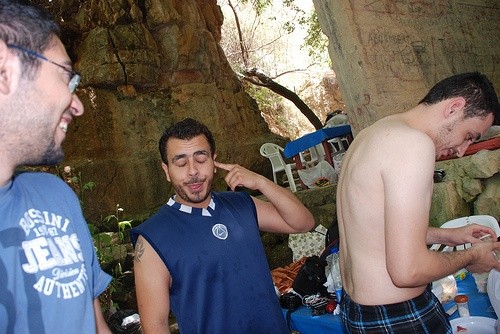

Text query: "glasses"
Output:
(7, 43), (80, 93)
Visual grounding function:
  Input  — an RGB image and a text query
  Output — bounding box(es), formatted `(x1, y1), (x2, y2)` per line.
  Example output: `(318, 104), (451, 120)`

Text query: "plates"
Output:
(440, 215), (500, 239)
(450, 316), (497, 334)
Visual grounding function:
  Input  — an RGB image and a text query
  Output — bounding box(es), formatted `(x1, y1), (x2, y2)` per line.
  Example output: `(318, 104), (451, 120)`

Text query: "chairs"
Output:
(260, 142), (303, 193)
(429, 214), (500, 252)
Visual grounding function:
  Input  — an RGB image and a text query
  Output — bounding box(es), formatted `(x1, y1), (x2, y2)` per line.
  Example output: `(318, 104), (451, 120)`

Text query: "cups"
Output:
(433, 170), (444, 182)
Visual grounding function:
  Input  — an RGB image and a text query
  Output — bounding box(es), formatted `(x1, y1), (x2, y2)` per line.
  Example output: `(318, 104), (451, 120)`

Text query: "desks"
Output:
(281, 249), (500, 334)
(284, 125), (354, 190)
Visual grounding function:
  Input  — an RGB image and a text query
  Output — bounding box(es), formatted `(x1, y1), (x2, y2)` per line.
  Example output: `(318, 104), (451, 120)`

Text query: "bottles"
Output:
(329, 248), (342, 302)
(455, 295), (469, 317)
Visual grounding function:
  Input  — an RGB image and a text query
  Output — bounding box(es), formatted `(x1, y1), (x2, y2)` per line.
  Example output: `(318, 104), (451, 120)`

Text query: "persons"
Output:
(0, 0), (113, 334)
(132, 120), (314, 334)
(335, 71), (500, 333)
